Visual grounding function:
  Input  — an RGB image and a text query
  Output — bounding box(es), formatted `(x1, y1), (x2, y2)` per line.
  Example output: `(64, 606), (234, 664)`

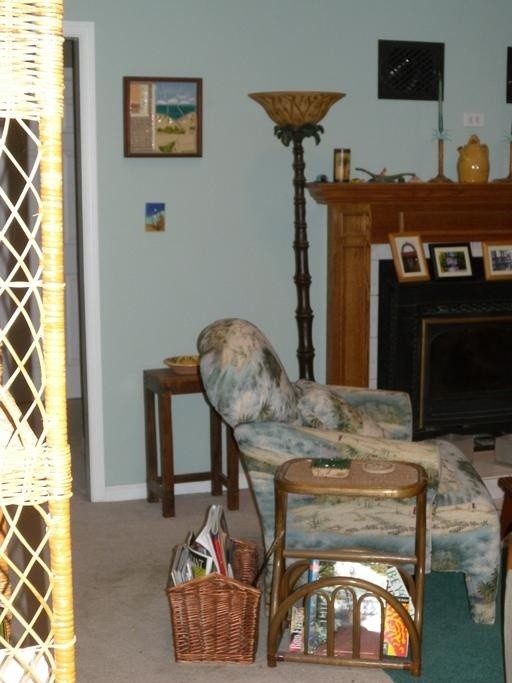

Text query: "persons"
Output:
(445, 251), (459, 271)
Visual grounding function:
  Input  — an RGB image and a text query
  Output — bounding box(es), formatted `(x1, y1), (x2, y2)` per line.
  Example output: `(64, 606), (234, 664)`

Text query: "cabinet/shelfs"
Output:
(265, 453), (430, 676)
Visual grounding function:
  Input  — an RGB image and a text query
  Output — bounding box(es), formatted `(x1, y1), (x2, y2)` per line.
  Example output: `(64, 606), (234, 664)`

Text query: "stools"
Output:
(142, 367), (240, 517)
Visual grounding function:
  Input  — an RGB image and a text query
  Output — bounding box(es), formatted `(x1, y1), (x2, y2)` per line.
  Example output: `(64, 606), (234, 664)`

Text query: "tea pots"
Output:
(456, 133), (491, 184)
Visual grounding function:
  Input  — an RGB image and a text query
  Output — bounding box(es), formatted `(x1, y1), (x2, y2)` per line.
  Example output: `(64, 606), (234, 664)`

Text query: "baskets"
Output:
(164, 536), (264, 664)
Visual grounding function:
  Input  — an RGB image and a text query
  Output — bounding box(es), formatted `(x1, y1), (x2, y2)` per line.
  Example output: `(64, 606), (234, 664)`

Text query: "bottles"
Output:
(333, 148), (351, 183)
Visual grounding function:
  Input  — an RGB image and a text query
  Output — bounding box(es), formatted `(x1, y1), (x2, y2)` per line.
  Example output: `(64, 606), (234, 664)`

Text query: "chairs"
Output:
(196, 316), (502, 625)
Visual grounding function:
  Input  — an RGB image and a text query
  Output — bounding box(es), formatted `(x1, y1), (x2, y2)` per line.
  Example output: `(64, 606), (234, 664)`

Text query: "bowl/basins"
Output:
(163, 356), (200, 376)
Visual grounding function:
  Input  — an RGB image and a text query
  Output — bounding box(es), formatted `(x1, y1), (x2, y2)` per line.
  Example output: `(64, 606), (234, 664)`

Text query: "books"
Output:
(170, 503), (242, 587)
(385, 566), (415, 620)
(288, 605), (306, 653)
(306, 558), (390, 659)
(382, 596), (411, 658)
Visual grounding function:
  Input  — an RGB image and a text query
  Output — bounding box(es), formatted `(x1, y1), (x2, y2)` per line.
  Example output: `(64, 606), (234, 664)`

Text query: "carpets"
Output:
(376, 573), (506, 683)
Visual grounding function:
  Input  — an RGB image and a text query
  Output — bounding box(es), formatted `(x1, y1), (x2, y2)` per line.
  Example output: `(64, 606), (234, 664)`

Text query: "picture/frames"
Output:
(387, 230), (511, 284)
(122, 75), (204, 158)
